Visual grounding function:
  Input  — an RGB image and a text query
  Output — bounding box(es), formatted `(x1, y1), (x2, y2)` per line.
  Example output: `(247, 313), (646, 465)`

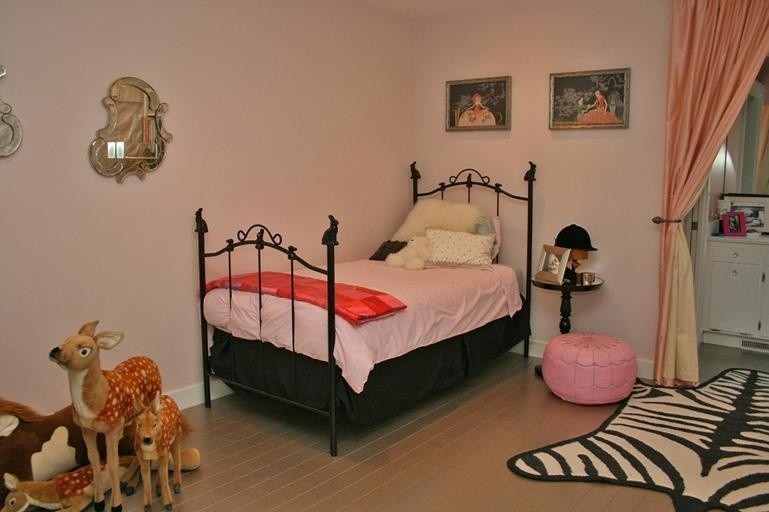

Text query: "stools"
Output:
(541, 332), (639, 406)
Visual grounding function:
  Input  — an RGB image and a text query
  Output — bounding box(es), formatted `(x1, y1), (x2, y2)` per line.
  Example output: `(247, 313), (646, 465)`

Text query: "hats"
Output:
(555, 224), (597, 250)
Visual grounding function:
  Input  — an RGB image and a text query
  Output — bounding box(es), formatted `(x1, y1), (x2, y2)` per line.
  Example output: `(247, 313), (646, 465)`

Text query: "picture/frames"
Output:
(445, 76), (511, 132)
(548, 66), (630, 129)
(718, 193), (769, 234)
(535, 243), (572, 284)
(723, 211), (746, 236)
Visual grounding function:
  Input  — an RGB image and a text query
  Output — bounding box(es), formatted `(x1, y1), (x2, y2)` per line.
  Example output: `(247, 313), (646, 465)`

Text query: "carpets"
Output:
(506, 368), (769, 512)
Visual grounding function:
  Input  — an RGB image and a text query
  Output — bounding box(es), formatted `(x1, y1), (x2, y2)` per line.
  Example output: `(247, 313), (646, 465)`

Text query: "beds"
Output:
(194, 156), (536, 457)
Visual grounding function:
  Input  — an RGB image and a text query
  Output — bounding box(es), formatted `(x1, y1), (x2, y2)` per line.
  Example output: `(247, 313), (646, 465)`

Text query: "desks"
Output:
(531, 273), (605, 375)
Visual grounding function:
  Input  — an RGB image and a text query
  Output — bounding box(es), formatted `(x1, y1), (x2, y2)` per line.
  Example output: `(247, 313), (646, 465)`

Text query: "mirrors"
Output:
(0, 65), (23, 157)
(87, 74), (171, 184)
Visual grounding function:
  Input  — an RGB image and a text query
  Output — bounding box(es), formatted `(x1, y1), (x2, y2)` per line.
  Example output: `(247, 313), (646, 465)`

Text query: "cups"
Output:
(582, 272), (592, 285)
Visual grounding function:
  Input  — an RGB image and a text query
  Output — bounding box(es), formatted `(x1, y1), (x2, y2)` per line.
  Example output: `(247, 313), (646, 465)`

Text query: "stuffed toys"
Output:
(0, 319), (201, 512)
(385, 235), (433, 270)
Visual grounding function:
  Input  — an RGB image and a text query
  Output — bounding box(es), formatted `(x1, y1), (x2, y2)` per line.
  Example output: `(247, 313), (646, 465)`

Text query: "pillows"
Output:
(424, 229), (494, 268)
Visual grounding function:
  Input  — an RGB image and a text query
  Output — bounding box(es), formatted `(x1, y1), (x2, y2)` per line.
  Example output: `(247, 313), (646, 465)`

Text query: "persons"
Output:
(458, 92), (496, 126)
(582, 90), (618, 123)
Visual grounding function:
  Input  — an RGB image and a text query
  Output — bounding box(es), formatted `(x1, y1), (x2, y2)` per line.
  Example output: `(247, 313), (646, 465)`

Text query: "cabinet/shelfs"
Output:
(704, 237), (769, 354)
